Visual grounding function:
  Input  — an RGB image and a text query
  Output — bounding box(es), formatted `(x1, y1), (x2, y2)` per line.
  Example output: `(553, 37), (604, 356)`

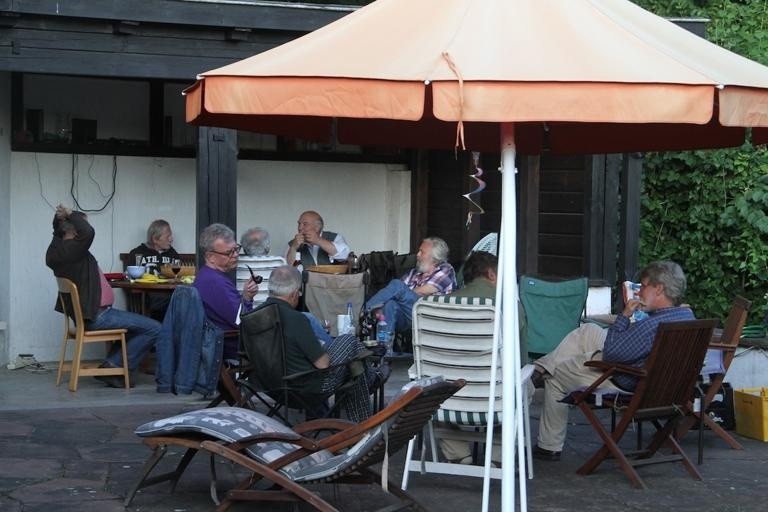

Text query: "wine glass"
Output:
(170, 258), (182, 282)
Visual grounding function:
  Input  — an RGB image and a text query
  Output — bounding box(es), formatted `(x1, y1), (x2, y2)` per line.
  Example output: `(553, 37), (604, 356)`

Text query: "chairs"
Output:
(228, 302), (394, 439)
(125, 374), (467, 510)
(56, 252), (753, 348)
(404, 294), (535, 512)
(56, 279), (134, 393)
(173, 285), (288, 423)
(658, 292), (754, 461)
(530, 315), (721, 492)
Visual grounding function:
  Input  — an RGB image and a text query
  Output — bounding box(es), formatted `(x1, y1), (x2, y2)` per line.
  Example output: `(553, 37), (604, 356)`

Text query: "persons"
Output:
(238, 226), (283, 259)
(286, 211), (351, 270)
(435, 250), (528, 465)
(193, 224), (258, 358)
(532, 261), (697, 462)
(364, 236), (457, 342)
(250, 264), (391, 431)
(45, 204), (162, 388)
(123, 219), (184, 351)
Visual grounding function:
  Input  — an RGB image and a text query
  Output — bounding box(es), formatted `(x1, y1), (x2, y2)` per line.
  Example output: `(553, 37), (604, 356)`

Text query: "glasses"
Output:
(209, 245), (242, 258)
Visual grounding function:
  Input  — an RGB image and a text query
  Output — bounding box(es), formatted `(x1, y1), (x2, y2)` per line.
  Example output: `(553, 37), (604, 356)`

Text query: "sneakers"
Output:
(369, 364), (391, 395)
(94, 366), (135, 387)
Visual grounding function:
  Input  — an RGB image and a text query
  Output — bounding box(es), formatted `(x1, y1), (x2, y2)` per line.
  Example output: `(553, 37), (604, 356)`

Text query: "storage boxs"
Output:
(734, 387), (768, 442)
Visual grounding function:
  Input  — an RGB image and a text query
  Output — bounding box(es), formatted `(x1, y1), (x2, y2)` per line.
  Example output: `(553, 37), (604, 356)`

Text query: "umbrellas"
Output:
(178, 1), (767, 512)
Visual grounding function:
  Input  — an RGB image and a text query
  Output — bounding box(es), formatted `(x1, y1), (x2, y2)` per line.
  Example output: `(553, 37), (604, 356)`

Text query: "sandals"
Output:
(442, 455), (474, 465)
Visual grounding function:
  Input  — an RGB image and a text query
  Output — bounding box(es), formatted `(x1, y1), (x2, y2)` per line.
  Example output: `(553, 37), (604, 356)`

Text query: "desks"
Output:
(364, 349), (414, 415)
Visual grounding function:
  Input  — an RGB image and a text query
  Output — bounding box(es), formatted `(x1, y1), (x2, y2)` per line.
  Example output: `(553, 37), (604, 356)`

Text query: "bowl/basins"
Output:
(160, 265), (195, 278)
(127, 265), (146, 279)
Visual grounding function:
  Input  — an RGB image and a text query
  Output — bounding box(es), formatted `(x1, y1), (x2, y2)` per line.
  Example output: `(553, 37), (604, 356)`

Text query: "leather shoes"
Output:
(525, 445), (562, 461)
(531, 369), (542, 388)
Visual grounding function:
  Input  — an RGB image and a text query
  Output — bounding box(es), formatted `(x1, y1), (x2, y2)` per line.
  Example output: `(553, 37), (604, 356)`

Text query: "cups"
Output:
(336, 315), (353, 337)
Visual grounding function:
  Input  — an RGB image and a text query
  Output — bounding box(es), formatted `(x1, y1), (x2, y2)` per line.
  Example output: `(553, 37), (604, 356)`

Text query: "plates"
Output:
(103, 273), (125, 279)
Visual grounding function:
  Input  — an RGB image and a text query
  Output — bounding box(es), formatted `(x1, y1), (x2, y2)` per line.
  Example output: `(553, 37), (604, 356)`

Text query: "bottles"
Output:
(352, 255), (358, 274)
(376, 314), (388, 349)
(343, 302), (356, 339)
(347, 251), (355, 274)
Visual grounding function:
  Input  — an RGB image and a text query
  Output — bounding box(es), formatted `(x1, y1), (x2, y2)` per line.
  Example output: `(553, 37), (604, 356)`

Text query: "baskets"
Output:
(303, 264), (349, 274)
